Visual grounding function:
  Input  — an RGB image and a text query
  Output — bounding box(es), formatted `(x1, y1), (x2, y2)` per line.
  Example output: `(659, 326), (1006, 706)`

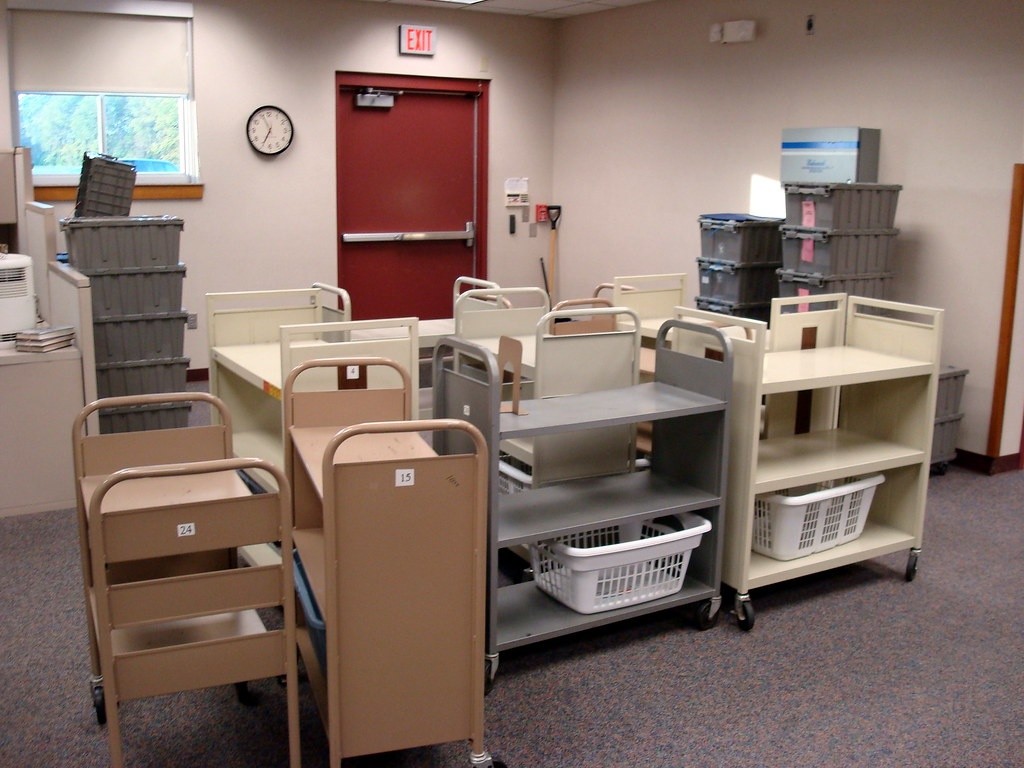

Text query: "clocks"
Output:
(246, 105), (295, 156)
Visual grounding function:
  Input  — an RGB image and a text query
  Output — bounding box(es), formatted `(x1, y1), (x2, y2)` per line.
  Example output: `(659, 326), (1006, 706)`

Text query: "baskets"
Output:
(751, 473), (885, 561)
(528, 512), (713, 615)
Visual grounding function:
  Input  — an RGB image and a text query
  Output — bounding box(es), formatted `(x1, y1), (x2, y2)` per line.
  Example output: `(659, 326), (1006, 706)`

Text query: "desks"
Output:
(0, 146), (98, 519)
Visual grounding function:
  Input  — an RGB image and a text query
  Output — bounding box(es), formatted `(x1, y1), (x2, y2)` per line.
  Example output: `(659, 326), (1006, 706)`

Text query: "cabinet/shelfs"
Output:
(70, 273), (945, 768)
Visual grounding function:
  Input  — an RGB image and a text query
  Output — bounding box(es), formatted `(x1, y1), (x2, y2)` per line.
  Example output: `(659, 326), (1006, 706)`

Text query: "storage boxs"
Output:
(462, 362), (532, 402)
(932, 364), (971, 471)
(528, 514), (714, 615)
(753, 474), (887, 562)
(498, 454), (650, 564)
(292, 545), (328, 688)
(696, 126), (903, 313)
(57, 153), (199, 434)
